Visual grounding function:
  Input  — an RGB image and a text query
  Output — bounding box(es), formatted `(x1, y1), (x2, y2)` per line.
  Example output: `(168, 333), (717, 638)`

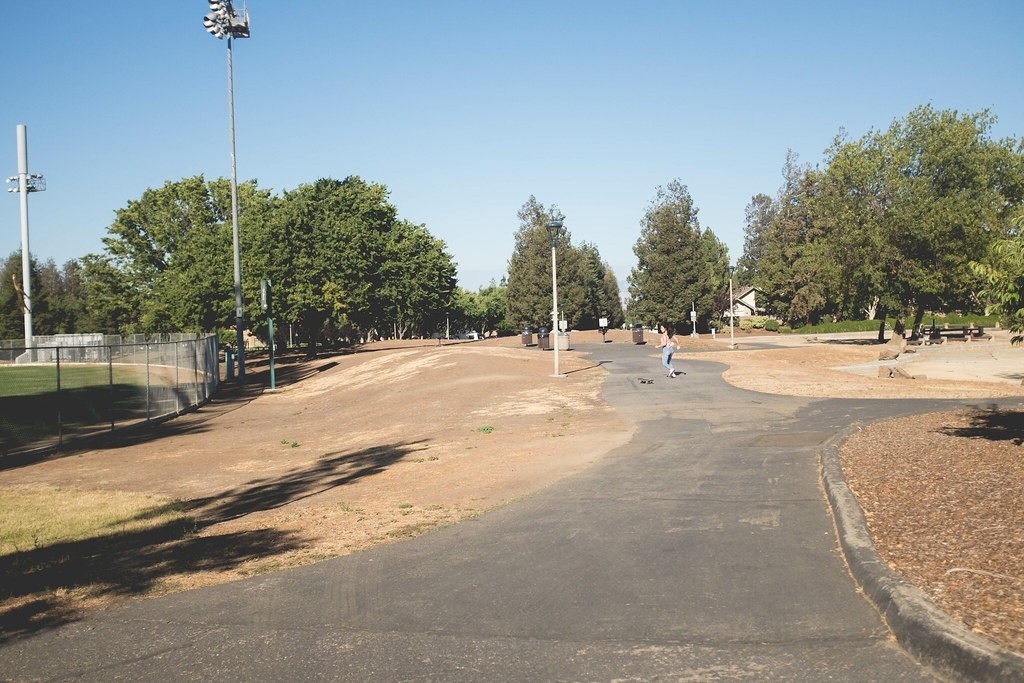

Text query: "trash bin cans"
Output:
(522, 325), (533, 347)
(537, 327), (549, 350)
(558, 332), (570, 351)
(631, 323), (643, 345)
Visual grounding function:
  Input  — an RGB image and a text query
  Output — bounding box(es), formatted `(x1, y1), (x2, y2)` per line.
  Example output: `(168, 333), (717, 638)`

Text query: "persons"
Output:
(655, 322), (680, 378)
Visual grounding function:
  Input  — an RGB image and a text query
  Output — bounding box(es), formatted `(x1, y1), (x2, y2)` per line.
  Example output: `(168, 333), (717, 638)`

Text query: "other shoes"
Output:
(668, 369), (675, 377)
(671, 372), (677, 378)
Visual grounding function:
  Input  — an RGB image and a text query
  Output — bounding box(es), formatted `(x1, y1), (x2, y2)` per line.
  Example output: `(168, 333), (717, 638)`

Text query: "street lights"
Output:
(6, 124), (43, 362)
(202, 0), (252, 383)
(726, 265), (736, 345)
(544, 220), (563, 377)
